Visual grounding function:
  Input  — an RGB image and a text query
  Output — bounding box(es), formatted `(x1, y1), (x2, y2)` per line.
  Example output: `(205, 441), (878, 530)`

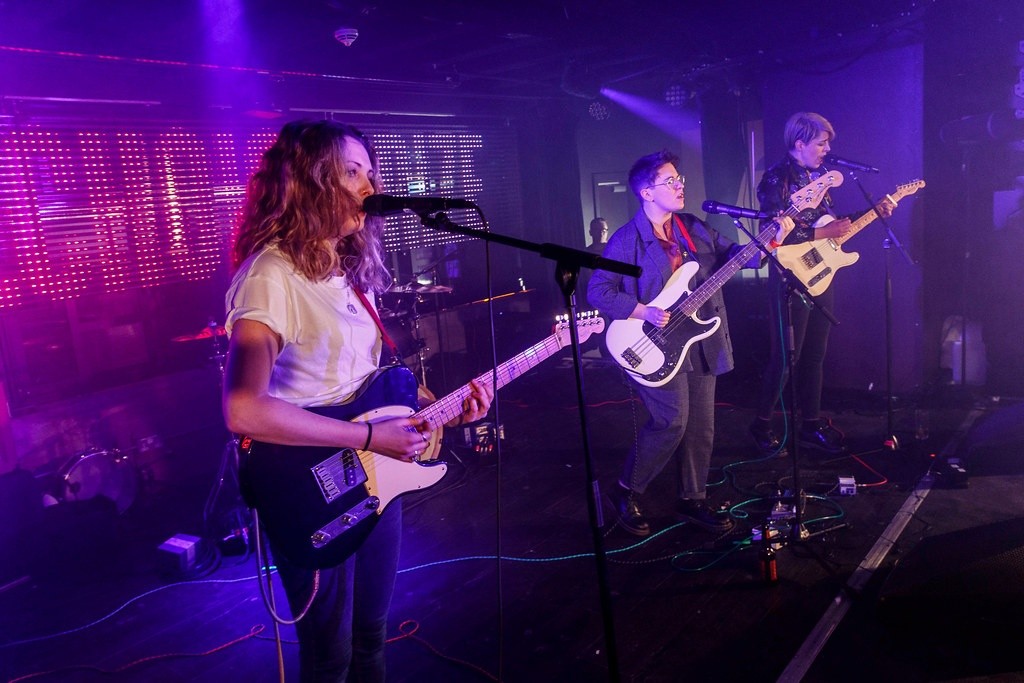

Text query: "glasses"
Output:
(591, 228), (608, 235)
(646, 173), (685, 191)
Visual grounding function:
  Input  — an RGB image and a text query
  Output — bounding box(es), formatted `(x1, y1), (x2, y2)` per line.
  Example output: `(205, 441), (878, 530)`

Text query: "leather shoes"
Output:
(602, 480), (650, 536)
(746, 423), (788, 457)
(670, 497), (732, 532)
(795, 428), (845, 452)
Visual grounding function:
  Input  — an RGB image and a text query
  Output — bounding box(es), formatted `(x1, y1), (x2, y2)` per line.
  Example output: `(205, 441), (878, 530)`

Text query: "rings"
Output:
(415, 451), (417, 455)
(420, 432), (427, 442)
(657, 321), (658, 323)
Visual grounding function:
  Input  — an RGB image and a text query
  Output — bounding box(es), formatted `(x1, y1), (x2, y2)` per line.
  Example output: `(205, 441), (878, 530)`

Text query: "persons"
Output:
(576, 217), (609, 303)
(750, 110), (895, 460)
(222, 118), (494, 683)
(586, 146), (796, 533)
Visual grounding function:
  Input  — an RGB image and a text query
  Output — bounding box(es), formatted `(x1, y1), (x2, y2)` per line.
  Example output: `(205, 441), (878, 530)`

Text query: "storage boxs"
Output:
(159, 534), (201, 582)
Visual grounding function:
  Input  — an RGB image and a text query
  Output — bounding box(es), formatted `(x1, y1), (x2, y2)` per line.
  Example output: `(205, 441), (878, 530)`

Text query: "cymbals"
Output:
(386, 283), (453, 294)
(168, 323), (227, 343)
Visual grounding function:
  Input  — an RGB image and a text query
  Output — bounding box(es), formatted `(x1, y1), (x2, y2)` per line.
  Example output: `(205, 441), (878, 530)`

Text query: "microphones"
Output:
(824, 153), (880, 173)
(701, 200), (772, 220)
(363, 194), (477, 217)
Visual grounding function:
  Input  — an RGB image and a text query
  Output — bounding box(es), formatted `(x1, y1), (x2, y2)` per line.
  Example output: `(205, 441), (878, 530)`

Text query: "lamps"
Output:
(586, 71), (756, 120)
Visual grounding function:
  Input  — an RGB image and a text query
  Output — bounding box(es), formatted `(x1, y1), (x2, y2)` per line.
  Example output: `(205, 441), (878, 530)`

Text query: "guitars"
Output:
(604, 168), (845, 388)
(774, 179), (926, 296)
(246, 311), (604, 578)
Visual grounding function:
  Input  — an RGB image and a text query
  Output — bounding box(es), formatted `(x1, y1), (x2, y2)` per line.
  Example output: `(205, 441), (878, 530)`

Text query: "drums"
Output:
(376, 307), (427, 367)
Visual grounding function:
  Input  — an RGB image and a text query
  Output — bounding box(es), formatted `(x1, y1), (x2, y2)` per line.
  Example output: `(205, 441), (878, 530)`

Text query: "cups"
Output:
(914, 409), (930, 440)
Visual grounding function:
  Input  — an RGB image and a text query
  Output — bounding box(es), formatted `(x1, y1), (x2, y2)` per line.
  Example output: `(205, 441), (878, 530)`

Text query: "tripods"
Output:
(730, 169), (930, 586)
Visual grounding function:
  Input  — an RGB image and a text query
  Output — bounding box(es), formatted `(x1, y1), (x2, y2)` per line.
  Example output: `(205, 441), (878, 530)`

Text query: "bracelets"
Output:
(362, 423), (372, 450)
(769, 240), (782, 249)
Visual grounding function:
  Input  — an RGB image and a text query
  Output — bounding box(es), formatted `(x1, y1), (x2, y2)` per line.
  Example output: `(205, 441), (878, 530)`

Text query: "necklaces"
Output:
(330, 272), (357, 314)
